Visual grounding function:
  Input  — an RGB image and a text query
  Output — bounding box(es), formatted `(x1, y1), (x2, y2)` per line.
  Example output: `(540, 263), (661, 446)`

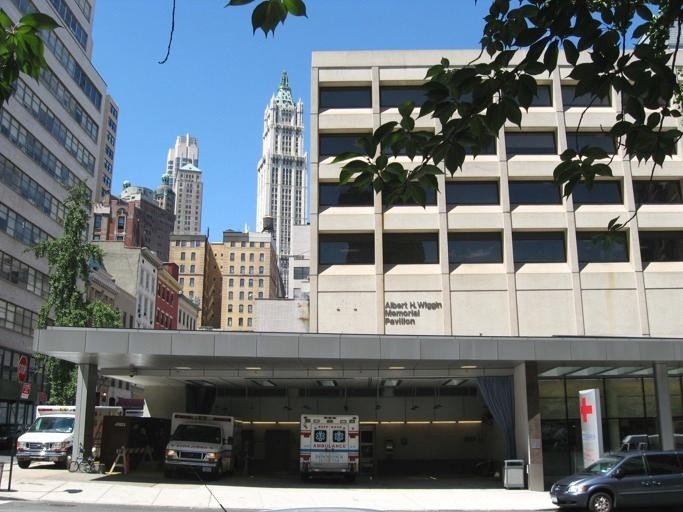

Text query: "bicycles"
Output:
(68, 447), (91, 472)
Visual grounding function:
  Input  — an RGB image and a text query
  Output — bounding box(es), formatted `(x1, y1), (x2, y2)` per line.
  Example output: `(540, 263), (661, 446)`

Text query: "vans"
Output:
(551, 449), (682, 512)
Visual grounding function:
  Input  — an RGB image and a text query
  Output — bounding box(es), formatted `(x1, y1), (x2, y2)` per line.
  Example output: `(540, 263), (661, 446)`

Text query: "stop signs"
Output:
(16, 355), (28, 383)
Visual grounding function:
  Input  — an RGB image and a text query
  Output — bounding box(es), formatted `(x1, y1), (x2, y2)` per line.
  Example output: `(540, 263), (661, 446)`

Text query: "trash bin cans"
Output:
(502, 459), (525, 489)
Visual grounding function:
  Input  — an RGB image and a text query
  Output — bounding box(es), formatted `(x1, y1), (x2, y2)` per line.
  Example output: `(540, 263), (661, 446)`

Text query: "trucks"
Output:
(16, 405), (123, 469)
(164, 412), (243, 480)
(299, 414), (360, 481)
(622, 435), (682, 452)
(361, 424), (376, 474)
